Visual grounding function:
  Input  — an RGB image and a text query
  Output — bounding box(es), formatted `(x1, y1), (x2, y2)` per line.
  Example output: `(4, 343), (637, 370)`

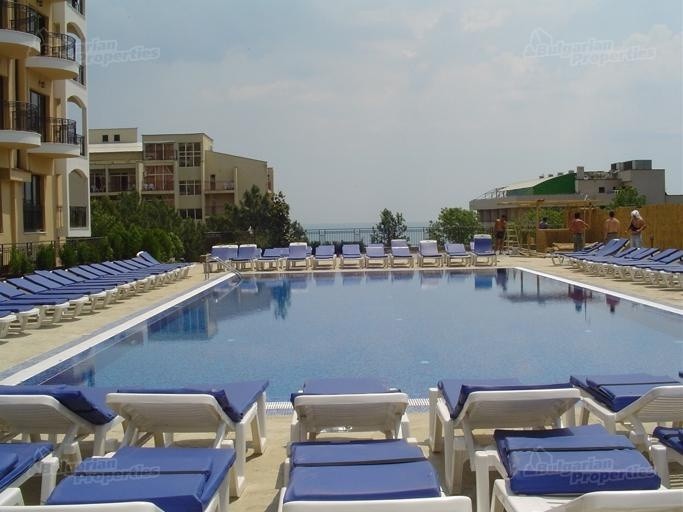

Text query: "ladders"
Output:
(503, 223), (521, 255)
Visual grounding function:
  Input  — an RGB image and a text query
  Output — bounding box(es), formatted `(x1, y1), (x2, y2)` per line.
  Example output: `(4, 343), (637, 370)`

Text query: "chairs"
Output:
(548, 237), (682, 292)
(0, 248), (193, 338)
(202, 233), (496, 272)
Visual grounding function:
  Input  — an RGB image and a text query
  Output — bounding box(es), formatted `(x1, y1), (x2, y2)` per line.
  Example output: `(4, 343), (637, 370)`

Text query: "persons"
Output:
(602, 210), (622, 243)
(495, 213), (505, 253)
(569, 212), (591, 251)
(494, 267), (510, 291)
(540, 217), (549, 230)
(567, 283), (594, 310)
(605, 295), (620, 312)
(624, 208), (646, 248)
